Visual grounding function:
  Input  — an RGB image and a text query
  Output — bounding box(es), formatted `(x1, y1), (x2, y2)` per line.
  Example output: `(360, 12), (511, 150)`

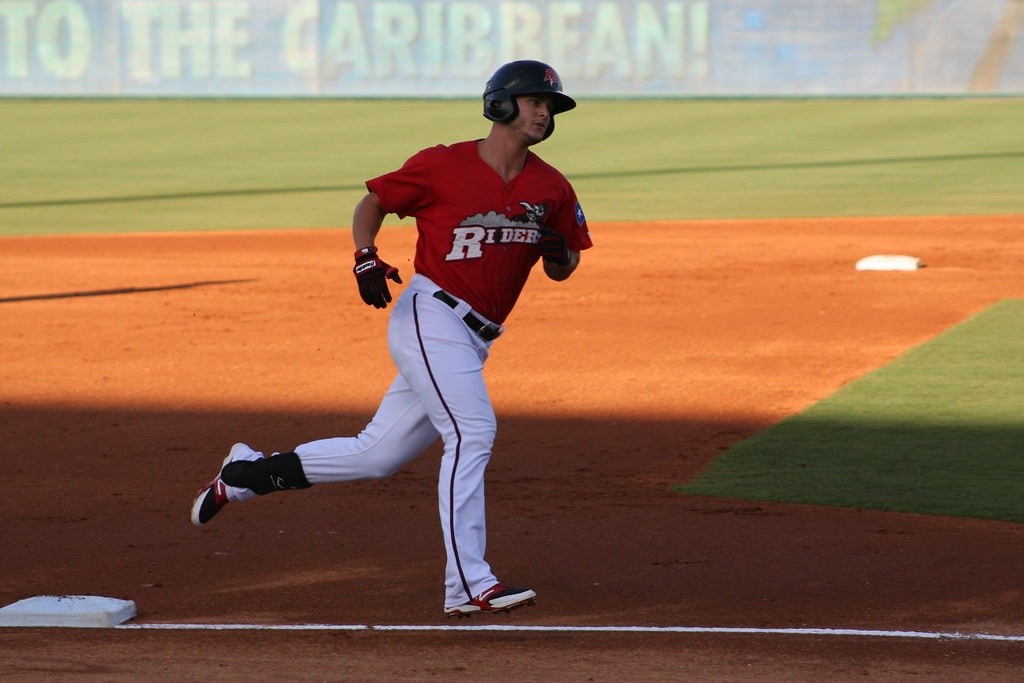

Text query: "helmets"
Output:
(483, 60), (576, 141)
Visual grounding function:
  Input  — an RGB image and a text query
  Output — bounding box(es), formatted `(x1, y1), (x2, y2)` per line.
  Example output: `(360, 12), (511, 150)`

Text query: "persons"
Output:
(189, 61), (593, 619)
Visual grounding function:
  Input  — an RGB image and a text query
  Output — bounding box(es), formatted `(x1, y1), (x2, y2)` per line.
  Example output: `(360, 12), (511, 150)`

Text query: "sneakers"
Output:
(445, 584), (537, 619)
(190, 442), (264, 528)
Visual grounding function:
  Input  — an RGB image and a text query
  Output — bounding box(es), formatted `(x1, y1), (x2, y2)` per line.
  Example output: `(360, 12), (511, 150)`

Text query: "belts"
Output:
(433, 291), (502, 341)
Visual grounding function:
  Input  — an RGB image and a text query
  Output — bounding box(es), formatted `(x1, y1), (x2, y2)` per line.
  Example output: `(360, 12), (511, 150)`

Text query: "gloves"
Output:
(537, 227), (571, 266)
(353, 246), (403, 309)
